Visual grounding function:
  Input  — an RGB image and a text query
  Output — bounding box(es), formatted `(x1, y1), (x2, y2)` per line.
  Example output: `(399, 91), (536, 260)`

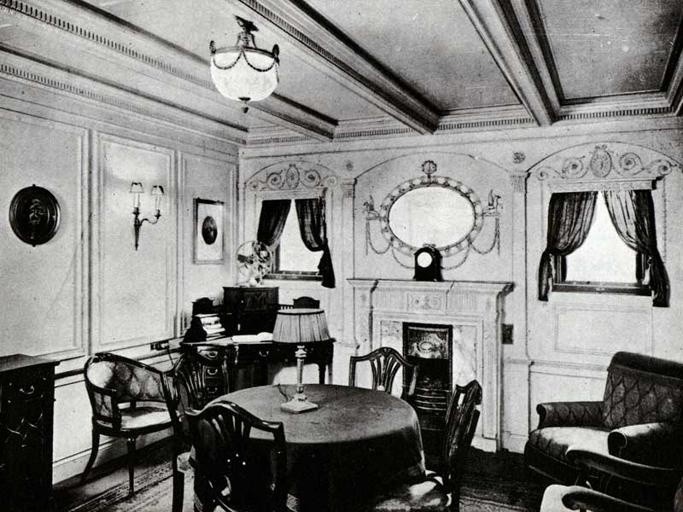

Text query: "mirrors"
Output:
(364, 160), (504, 271)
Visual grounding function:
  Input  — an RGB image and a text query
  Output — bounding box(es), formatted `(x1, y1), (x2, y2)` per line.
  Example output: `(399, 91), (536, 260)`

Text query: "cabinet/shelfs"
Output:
(0, 354), (60, 512)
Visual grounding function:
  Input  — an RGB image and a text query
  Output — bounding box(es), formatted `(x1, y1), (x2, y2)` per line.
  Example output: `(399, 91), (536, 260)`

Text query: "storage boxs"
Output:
(223, 287), (278, 313)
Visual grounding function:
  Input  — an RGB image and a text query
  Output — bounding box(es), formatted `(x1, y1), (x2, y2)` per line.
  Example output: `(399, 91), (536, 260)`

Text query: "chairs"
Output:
(174, 352), (226, 410)
(349, 347), (419, 407)
(184, 400), (288, 512)
(372, 379), (483, 512)
(81, 352), (184, 512)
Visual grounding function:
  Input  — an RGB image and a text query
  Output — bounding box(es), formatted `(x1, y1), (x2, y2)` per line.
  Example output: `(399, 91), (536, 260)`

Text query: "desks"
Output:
(179, 335), (337, 392)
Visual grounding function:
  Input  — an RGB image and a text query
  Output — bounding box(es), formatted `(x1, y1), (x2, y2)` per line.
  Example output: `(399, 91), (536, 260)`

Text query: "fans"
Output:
(235, 241), (273, 288)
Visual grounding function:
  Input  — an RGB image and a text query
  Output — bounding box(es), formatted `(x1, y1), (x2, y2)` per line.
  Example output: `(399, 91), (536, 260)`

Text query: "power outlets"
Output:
(151, 340), (169, 350)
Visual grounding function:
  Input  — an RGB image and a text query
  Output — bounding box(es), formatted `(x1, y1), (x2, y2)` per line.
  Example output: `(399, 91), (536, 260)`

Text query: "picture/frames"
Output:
(192, 198), (225, 265)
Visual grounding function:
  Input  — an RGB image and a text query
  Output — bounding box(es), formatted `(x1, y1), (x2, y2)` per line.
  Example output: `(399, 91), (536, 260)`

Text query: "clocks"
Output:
(413, 247), (445, 282)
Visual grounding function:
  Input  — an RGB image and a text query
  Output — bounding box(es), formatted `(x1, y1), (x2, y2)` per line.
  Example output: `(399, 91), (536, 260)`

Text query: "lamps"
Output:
(209, 18), (280, 114)
(272, 308), (331, 414)
(129, 181), (164, 251)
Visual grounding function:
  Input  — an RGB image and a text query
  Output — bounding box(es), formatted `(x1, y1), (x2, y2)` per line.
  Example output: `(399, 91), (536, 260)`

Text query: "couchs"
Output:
(523, 352), (682, 512)
